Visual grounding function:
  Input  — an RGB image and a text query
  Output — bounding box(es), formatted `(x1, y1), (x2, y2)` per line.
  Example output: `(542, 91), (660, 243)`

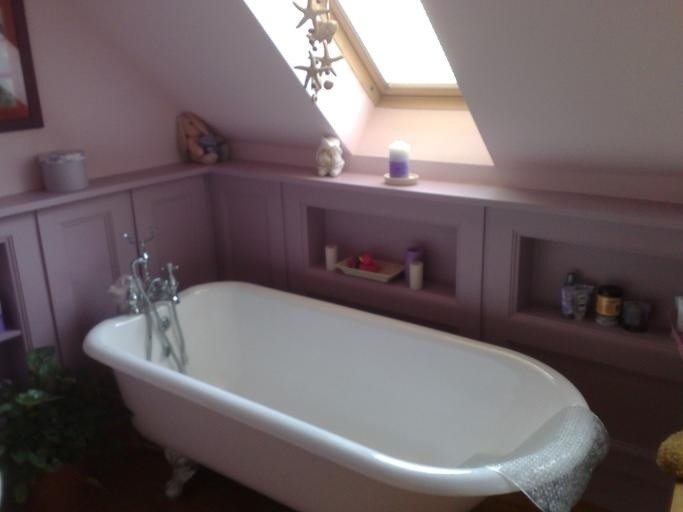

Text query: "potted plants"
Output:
(0, 345), (135, 512)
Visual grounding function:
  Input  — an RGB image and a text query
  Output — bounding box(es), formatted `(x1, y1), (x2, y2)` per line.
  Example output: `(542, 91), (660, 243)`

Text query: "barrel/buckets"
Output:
(37, 147), (86, 191)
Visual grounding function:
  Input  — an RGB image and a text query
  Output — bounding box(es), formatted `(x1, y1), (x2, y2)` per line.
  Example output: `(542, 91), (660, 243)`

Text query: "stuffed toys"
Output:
(175, 113), (228, 164)
(315, 137), (346, 176)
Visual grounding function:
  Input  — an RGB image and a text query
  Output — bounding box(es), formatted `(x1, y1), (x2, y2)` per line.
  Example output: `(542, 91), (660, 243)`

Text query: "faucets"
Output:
(147, 262), (182, 304)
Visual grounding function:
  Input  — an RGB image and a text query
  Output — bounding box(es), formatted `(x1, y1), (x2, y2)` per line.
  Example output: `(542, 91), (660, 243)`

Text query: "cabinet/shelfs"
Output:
(283, 185), (484, 333)
(209, 174), (288, 292)
(34, 178), (217, 393)
(485, 209), (683, 382)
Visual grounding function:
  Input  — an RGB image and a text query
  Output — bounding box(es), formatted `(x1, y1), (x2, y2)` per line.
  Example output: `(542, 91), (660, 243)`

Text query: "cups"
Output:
(325, 243), (339, 272)
(404, 247), (425, 291)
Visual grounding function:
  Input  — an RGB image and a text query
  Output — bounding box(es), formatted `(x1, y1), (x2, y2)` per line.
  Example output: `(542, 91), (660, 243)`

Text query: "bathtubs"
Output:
(82, 281), (597, 512)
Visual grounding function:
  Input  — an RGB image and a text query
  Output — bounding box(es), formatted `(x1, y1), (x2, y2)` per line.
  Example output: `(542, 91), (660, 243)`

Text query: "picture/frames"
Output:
(0, 1), (44, 139)
